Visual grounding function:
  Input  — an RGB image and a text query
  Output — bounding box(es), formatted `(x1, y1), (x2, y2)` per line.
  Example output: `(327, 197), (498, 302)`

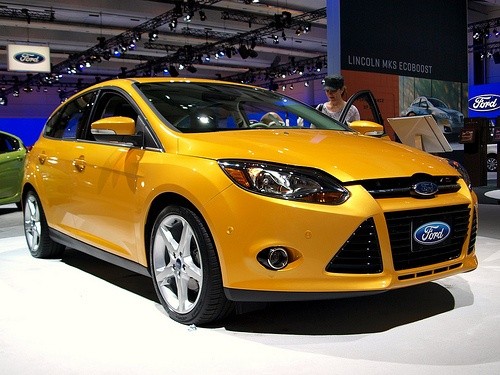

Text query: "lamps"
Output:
(86, 11), (322, 91)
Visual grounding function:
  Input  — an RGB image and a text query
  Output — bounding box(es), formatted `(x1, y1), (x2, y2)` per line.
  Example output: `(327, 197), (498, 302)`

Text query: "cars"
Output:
(21, 77), (478, 327)
(487, 143), (498, 171)
(435, 118), (455, 135)
(408, 96), (466, 130)
(1, 131), (29, 212)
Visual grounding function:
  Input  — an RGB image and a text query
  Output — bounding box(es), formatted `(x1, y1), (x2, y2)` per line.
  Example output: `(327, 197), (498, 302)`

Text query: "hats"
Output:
(323, 75), (345, 92)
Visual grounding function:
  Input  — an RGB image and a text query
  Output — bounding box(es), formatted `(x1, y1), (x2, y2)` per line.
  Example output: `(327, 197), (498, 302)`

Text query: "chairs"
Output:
(190, 108), (219, 129)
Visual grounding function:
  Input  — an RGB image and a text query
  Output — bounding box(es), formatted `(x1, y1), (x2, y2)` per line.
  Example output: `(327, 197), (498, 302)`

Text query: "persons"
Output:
(309, 76), (362, 132)
